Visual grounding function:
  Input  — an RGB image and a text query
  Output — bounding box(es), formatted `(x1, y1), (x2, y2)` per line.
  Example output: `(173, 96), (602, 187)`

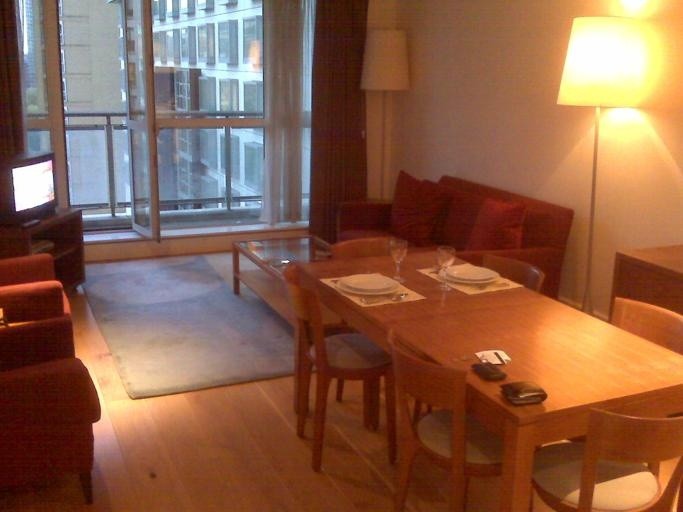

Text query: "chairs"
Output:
(0, 252), (101, 499)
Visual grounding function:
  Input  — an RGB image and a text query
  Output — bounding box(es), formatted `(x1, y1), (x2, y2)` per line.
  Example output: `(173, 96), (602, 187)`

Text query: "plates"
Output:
(337, 275), (393, 295)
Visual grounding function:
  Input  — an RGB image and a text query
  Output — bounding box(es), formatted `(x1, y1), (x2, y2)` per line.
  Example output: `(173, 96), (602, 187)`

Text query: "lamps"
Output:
(361, 28), (410, 200)
(558, 14), (641, 312)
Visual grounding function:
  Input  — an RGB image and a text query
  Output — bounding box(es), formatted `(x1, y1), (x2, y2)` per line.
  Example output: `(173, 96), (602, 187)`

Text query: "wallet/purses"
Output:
(499, 381), (548, 406)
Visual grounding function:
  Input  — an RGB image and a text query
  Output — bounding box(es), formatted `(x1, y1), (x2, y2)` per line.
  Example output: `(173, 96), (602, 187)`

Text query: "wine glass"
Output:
(436, 246), (455, 291)
(389, 239), (408, 283)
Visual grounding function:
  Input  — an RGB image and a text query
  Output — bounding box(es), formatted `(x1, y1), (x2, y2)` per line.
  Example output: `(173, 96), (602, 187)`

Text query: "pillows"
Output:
(391, 171), (451, 247)
(468, 199), (529, 250)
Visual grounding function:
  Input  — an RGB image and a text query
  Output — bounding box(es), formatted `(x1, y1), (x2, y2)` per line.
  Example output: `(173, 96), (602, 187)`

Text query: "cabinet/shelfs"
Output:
(0, 208), (86, 293)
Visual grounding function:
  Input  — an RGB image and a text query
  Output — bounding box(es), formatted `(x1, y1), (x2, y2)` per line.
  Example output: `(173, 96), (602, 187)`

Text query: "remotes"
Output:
(21, 219), (42, 226)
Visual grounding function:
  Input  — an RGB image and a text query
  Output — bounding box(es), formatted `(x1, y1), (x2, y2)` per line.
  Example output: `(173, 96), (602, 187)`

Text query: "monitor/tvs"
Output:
(3, 151), (59, 226)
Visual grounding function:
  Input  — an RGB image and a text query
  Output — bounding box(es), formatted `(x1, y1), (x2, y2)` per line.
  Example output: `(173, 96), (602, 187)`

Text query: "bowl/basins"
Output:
(441, 267), (499, 286)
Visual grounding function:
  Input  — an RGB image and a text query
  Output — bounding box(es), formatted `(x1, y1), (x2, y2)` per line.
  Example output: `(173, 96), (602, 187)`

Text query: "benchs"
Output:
(336, 174), (575, 299)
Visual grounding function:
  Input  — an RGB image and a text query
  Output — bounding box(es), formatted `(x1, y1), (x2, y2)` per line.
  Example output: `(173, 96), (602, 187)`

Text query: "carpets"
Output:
(82, 248), (318, 400)
